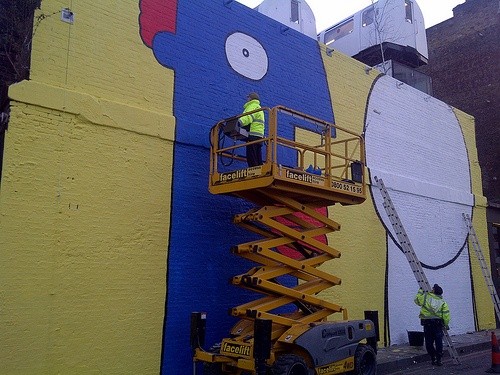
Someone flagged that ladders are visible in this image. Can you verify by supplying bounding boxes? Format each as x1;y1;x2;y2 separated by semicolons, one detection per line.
374;176;462;365
462;213;500;322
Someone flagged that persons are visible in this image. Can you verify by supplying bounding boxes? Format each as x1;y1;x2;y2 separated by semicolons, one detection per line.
237;93;265;167
413;284;451;366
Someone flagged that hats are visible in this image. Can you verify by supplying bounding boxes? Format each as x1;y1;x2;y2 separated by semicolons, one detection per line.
434;284;443;295
247;91;258;99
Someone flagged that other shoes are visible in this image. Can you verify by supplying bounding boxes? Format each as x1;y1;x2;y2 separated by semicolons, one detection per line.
432;357;444;365
